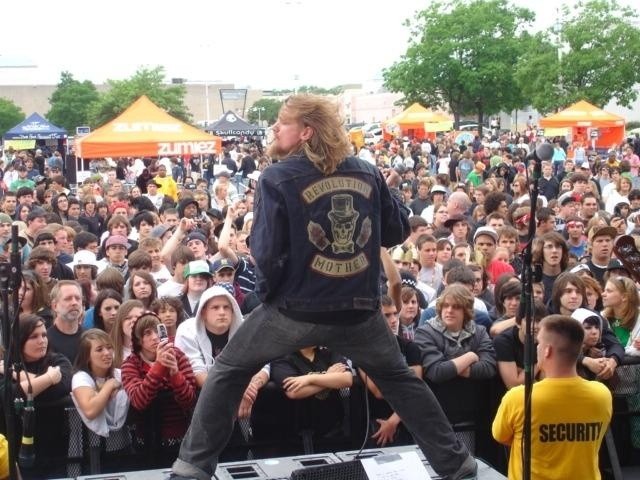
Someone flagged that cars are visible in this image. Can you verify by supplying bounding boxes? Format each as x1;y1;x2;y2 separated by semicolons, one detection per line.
359;118;390;149
454;120;495;135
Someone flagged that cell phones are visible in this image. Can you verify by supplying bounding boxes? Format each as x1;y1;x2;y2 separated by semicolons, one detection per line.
158;323;169;343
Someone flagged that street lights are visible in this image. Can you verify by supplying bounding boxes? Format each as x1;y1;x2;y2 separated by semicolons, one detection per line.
251;104;266;127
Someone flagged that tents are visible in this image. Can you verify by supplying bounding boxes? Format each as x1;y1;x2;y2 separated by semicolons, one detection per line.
530;101;629;154
9;107;69;143
74;97;216;162
375;99;458;142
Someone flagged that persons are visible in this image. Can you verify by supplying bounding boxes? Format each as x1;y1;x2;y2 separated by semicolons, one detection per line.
166;95;482;479
1;116;639;440
490;312;611;479
0;313;70;479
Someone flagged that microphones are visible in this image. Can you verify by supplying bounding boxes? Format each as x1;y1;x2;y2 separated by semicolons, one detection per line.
19;386;36;467
526;143;554;160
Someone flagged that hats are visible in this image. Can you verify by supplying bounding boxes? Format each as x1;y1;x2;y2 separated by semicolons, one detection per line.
472;225;499;242
179;197;199;216
588;223;617;243
183;259;214;278
185;231;208;244
104;235;128;251
71;250;99;269
211;258;235;273
